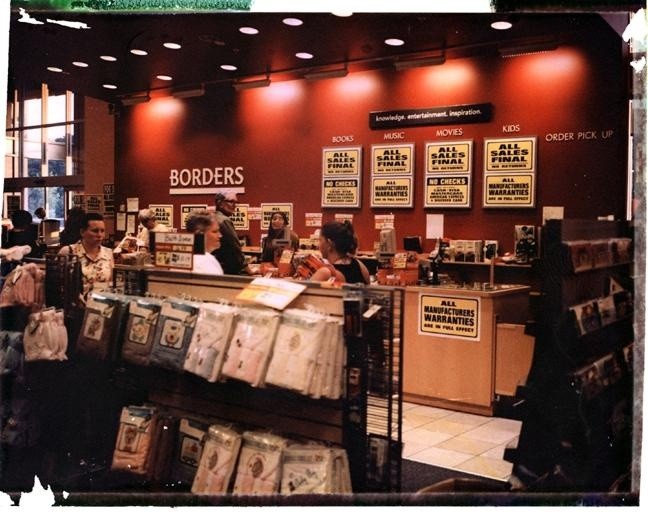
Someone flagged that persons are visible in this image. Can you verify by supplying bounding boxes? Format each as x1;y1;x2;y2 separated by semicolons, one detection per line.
34;209;50;224
181;208;225;274
310;217;371;287
260;209;299;263
137;206;170;244
52;213;114;293
212;188;254;275
9;208;45;260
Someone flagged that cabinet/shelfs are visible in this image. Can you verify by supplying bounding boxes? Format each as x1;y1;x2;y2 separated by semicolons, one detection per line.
502;216;635;493
